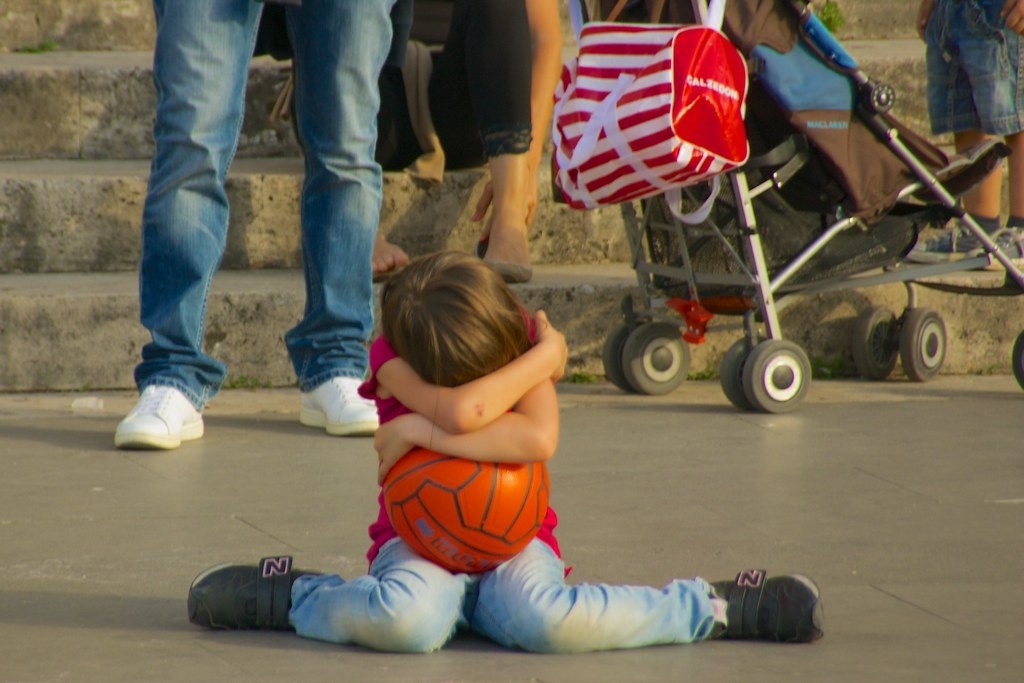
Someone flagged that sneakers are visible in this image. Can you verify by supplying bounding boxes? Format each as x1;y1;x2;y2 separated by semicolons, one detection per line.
297;373;380;438
906;219;989;263
113;384;205;449
982;225;1024;272
710;568;826;644
190;552;321;636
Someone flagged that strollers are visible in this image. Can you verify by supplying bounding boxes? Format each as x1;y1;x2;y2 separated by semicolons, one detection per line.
570;0;1024;415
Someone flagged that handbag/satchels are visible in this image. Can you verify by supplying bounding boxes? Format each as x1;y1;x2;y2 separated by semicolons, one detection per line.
549;23;749;225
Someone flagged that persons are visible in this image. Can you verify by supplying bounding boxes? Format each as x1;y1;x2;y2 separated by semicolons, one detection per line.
270;0;561;281
906;0;1024;271
187;250;824;652
113;1;394;450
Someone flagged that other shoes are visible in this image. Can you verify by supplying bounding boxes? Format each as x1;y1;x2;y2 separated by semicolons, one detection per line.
483;254;532;284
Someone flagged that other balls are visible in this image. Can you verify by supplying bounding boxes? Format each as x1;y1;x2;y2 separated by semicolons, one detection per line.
382;445;550;574
